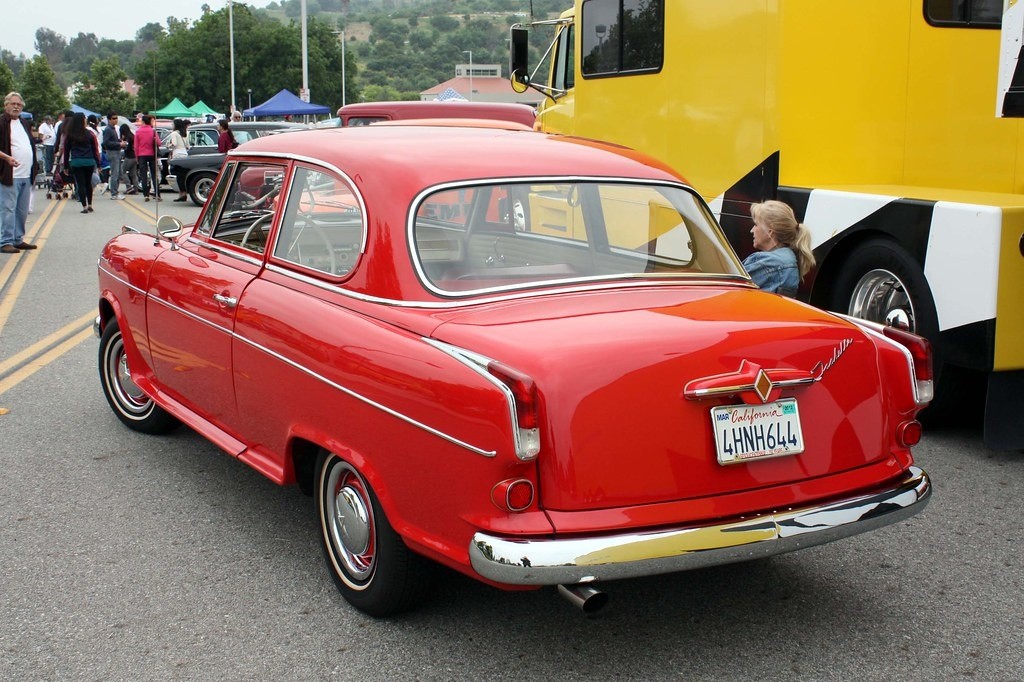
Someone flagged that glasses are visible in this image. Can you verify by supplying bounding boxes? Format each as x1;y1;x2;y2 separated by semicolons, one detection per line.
111;117;118;120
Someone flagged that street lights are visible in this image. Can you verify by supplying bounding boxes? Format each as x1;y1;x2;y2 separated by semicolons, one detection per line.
462;50;472;99
331;31;346;107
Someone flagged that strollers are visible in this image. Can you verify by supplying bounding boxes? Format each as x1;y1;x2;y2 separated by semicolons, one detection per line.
47;159;76;201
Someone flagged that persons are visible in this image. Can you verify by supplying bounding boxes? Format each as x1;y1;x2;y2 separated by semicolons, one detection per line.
21;91;293;213
688;199;815;301
0;92;37;254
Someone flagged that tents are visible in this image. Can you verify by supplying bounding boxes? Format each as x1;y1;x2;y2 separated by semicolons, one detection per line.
20;88;333;125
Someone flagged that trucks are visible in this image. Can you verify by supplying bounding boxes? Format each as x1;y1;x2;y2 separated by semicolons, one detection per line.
509;0;1023;439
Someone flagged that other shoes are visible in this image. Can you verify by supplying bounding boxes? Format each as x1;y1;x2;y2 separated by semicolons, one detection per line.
101;183;108;195
145;196;150;202
80;209;88;214
156;197;162;201
124;188;134;194
14;242;37;250
1;245;20;253
172;194;187;201
118;194;125;200
88;207;94;212
132;190;139;195
110;194;118;201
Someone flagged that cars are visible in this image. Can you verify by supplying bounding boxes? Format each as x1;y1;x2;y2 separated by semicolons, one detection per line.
94;120;933;620
127;99;535;231
